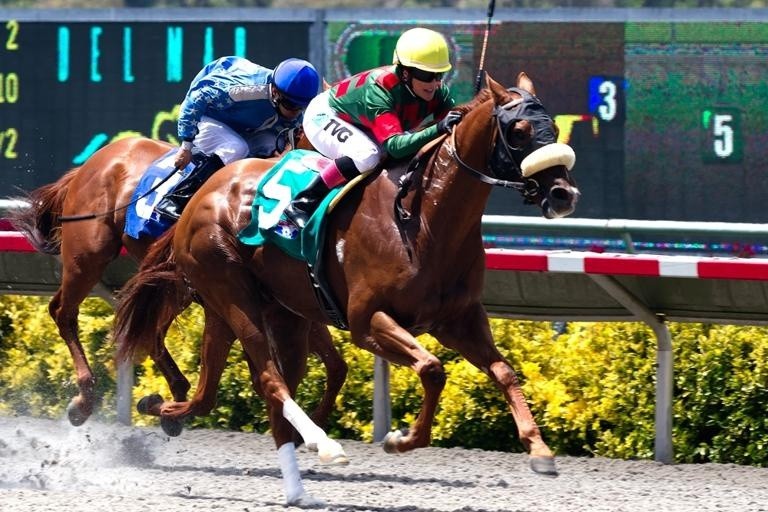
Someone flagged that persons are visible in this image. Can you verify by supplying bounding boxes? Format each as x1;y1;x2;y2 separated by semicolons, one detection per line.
283;28;462;231
154;56;319;220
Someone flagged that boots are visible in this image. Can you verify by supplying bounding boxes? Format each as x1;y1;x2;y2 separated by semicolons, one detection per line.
153;152;225;220
283;158;348;231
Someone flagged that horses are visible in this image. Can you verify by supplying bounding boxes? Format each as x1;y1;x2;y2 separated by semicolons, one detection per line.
106;70;582;508
0;76;348;450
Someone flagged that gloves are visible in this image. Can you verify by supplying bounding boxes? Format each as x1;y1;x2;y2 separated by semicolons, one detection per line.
437;110;462;134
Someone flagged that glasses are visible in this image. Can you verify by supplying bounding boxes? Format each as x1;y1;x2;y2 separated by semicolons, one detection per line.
279;93;301;111
407;68;443;82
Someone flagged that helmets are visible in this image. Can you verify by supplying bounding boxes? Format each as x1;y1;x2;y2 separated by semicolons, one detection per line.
392;28;452;73
272;58;323;105
